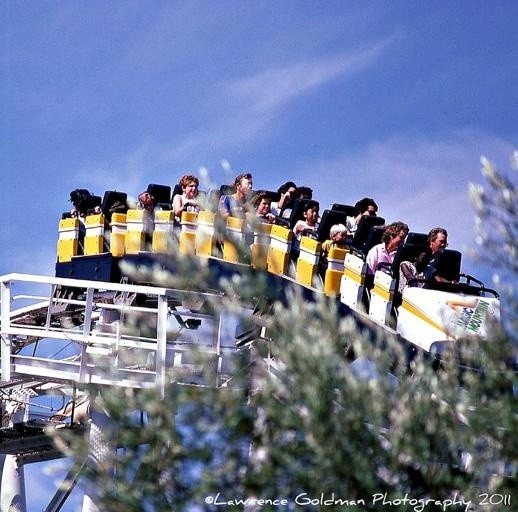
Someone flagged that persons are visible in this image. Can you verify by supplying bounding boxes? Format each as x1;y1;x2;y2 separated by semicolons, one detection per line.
70;173;459;295
